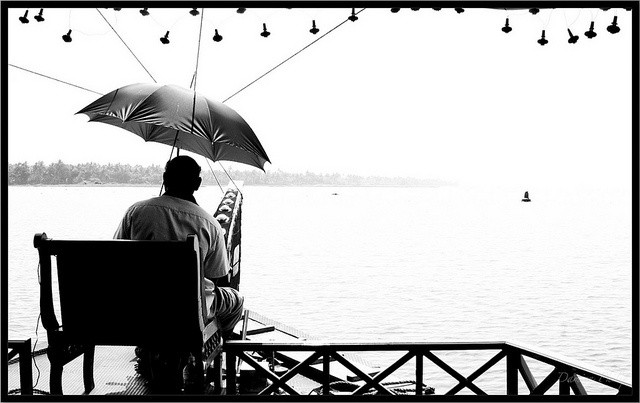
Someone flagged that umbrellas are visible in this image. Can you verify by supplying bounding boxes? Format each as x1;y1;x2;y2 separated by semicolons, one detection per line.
74;70;272;197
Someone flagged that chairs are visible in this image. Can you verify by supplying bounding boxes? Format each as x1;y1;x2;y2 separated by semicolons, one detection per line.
33;229;226;397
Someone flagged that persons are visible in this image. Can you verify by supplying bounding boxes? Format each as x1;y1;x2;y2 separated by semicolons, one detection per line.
112;155;244;330
